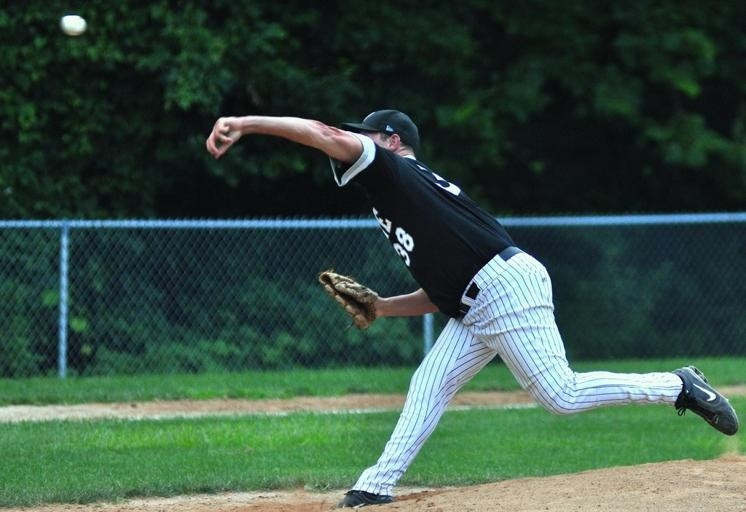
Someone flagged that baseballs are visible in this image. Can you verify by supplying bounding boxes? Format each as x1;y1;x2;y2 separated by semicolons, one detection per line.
58;14;87;35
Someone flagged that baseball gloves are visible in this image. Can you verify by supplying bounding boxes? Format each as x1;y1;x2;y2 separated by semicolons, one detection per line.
317;271;378;330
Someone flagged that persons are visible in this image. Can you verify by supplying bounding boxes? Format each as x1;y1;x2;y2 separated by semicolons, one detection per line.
203;107;742;510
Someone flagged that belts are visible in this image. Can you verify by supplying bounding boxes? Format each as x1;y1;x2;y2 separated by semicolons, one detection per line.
458;246;523;312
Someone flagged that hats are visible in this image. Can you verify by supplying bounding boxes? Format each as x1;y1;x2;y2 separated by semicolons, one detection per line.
341;110;419;145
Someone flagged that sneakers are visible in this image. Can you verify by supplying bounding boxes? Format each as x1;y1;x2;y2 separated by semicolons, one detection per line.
672;365;738;435
338;490;394;508
